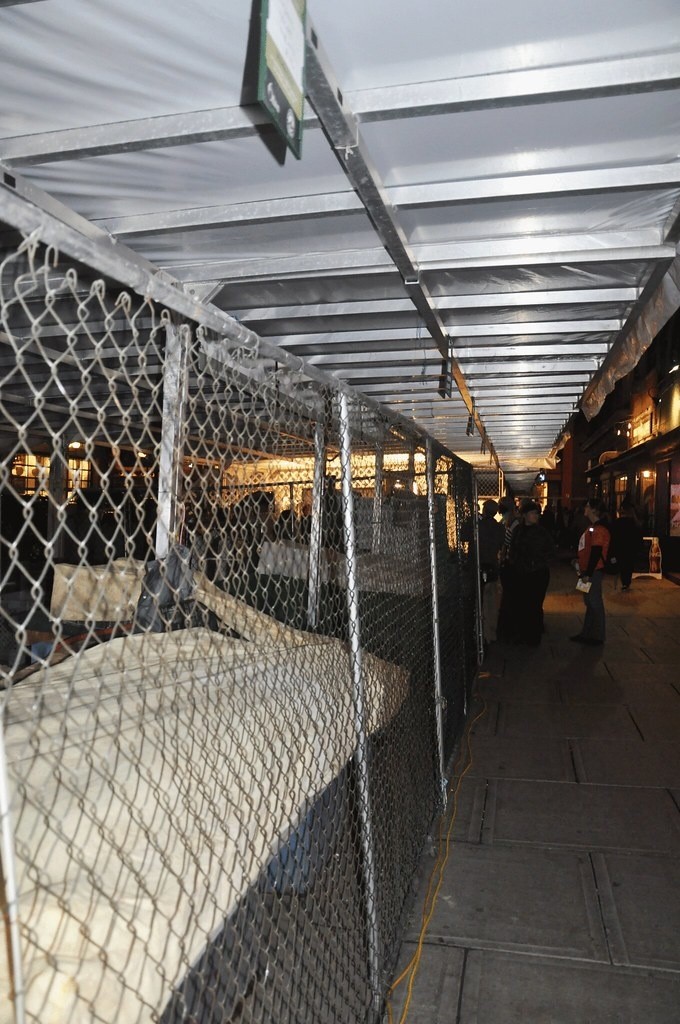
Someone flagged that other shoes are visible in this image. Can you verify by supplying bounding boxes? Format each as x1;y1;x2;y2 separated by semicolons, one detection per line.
621;584;629;593
568;632;605;651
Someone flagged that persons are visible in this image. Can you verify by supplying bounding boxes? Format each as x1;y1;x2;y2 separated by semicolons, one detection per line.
494;495;520;589
614;502;643;592
566;496;612;647
472;499;504;644
536;491;590;555
509;500;554;652
108;426;470;721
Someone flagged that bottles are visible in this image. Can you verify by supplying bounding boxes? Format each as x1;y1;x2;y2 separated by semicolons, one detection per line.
648;536;663;573
